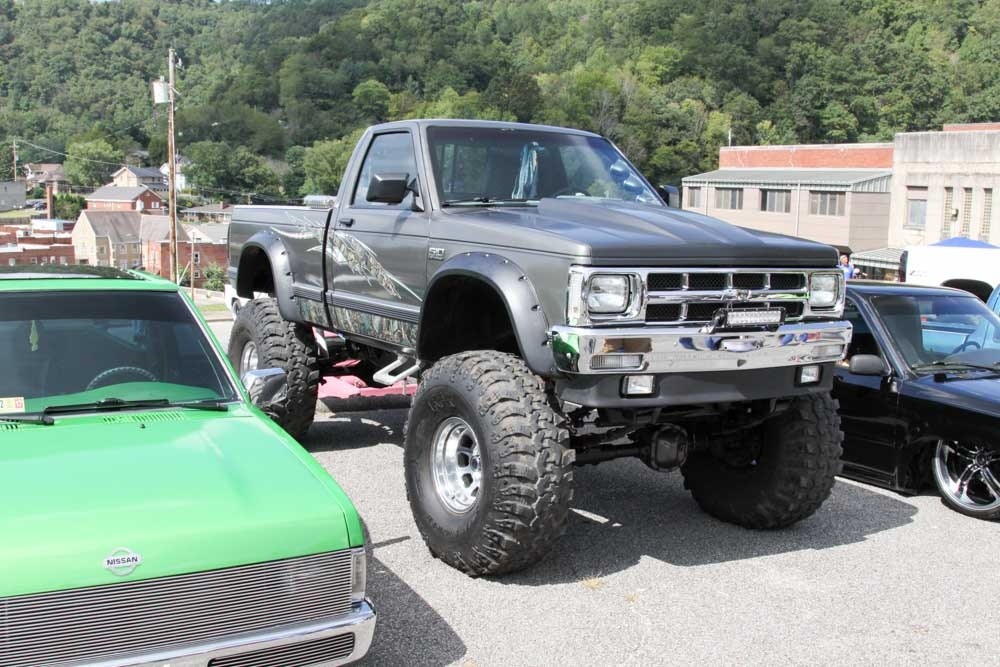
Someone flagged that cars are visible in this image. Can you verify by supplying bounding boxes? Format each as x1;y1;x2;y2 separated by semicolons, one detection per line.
825;281;1000;519
1;262;379;665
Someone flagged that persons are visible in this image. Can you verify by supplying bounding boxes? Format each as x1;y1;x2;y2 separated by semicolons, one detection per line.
840;255;853;280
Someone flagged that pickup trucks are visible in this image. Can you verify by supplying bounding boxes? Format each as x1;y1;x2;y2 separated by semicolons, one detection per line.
920;284;1000;357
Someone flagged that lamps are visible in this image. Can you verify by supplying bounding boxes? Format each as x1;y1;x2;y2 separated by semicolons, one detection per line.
951;208;958;215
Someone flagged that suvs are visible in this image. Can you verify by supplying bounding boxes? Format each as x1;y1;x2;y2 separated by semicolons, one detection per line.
217;116;859;581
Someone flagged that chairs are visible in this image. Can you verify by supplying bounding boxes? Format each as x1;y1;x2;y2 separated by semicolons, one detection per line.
843;317;882;362
38;334;124;396
